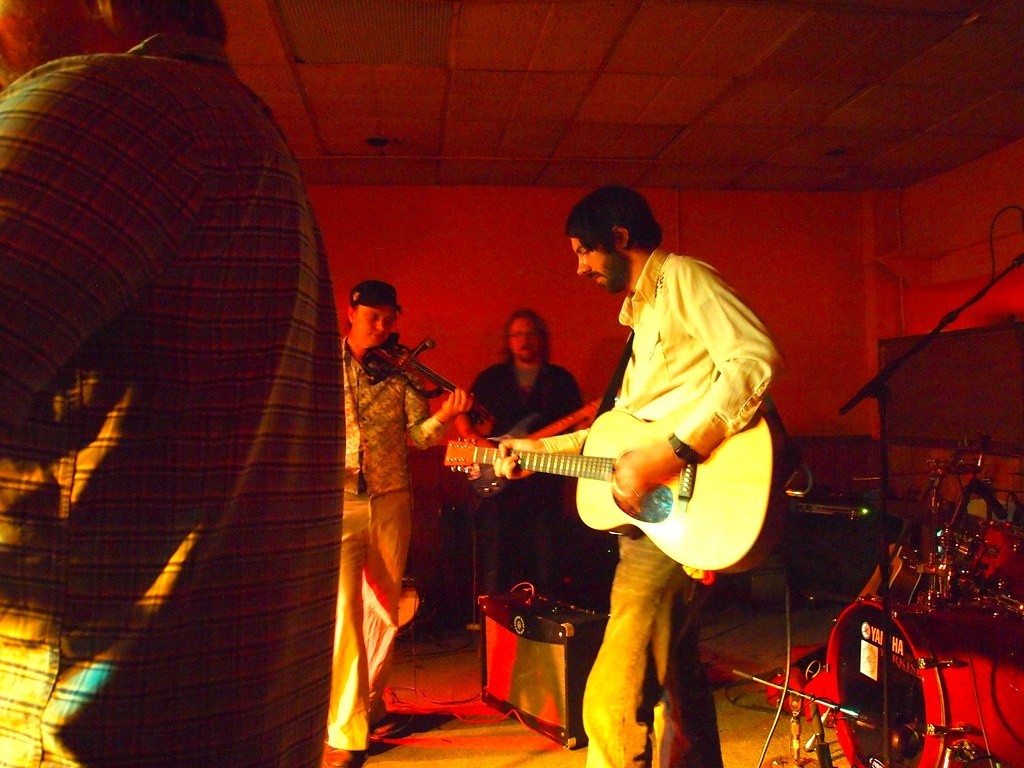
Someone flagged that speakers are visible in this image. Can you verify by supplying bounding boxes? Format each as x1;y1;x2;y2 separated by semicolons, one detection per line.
475;593;608;751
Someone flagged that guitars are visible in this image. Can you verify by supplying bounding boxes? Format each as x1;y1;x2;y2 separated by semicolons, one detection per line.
854;448;955;606
442;397;788;575
464;396;604;499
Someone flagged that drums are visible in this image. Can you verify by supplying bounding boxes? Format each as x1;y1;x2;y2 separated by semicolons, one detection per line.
822;511;1023;768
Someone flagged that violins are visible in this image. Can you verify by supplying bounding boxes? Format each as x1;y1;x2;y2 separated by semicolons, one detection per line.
370;341;496;437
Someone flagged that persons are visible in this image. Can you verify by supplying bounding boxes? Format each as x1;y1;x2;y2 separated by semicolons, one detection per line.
456;309;584;592
318;279;475;768
492;186;784;768
1;0;349;768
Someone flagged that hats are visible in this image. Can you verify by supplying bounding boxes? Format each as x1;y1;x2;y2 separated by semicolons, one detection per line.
350;281;402;312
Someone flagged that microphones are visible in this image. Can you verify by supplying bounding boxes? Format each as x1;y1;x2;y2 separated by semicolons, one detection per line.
887;723;920;760
977;482;1009;520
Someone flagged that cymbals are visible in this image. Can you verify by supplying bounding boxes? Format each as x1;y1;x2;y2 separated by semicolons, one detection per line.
951;445;1023;460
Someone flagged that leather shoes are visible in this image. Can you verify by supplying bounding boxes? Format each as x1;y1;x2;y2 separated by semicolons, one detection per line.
324;743;357;768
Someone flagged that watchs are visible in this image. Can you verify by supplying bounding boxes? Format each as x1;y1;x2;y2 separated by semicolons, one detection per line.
667;433;699;464
